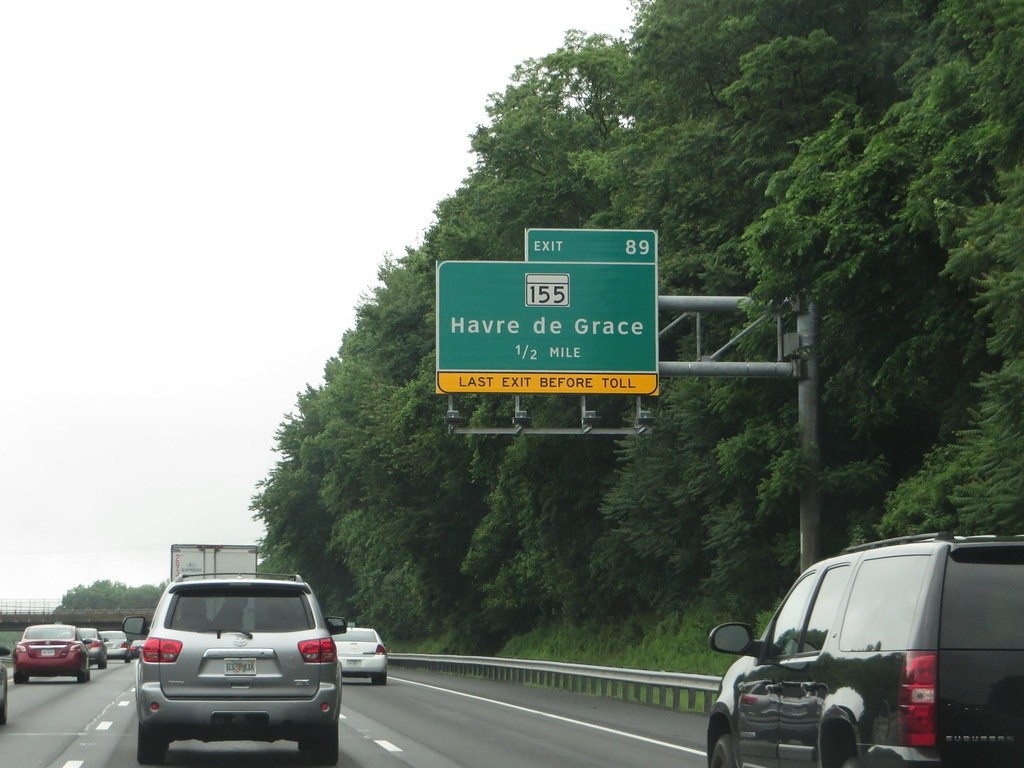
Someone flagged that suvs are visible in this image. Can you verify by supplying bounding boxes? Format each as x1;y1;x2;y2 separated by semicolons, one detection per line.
701;527;1024;768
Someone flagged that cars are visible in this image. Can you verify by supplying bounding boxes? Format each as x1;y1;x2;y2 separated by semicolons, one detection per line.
97;630;129;661
122;572;345;767
125;640;148;664
330;626;387;686
11;624;89;684
0;645;11;726
74;627;110;669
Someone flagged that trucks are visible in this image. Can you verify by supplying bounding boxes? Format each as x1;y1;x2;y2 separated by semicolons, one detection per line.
170;543;258;587
